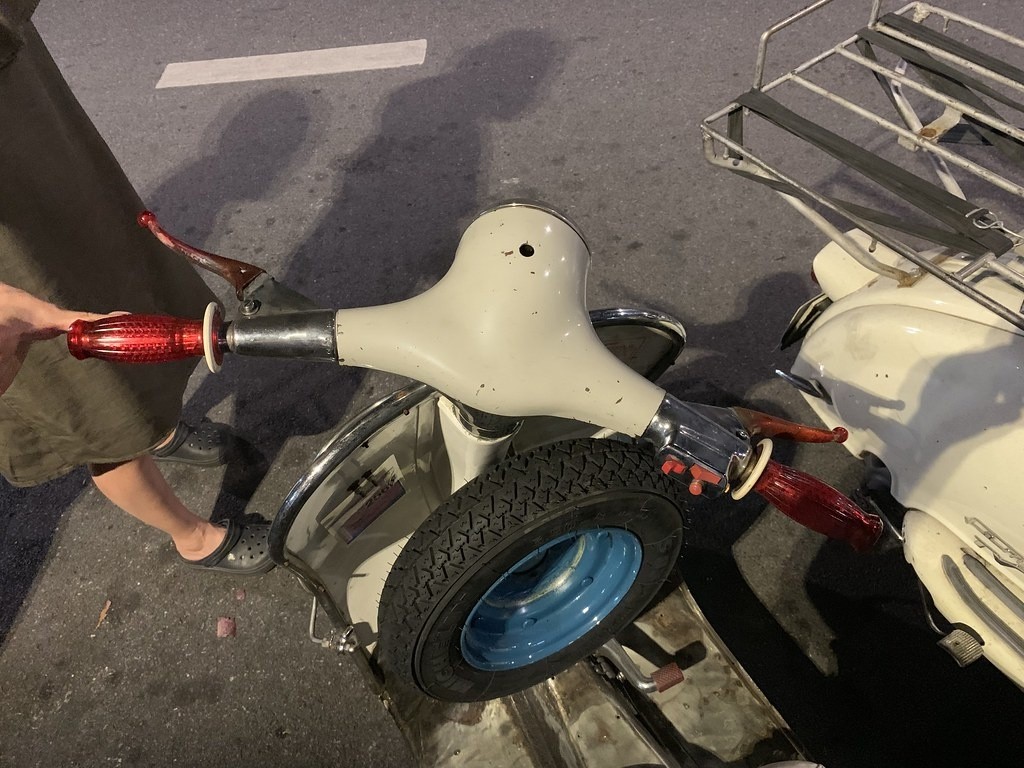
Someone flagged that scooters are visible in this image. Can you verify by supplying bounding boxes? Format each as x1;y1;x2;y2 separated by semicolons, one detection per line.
65;204;888;768
698;0;1024;692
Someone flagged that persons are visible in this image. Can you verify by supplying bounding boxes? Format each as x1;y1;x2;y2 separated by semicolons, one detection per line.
0;0;277;576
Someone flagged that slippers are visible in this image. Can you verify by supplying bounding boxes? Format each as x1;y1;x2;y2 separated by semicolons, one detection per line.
147;419;233;468
177;518;278;574
